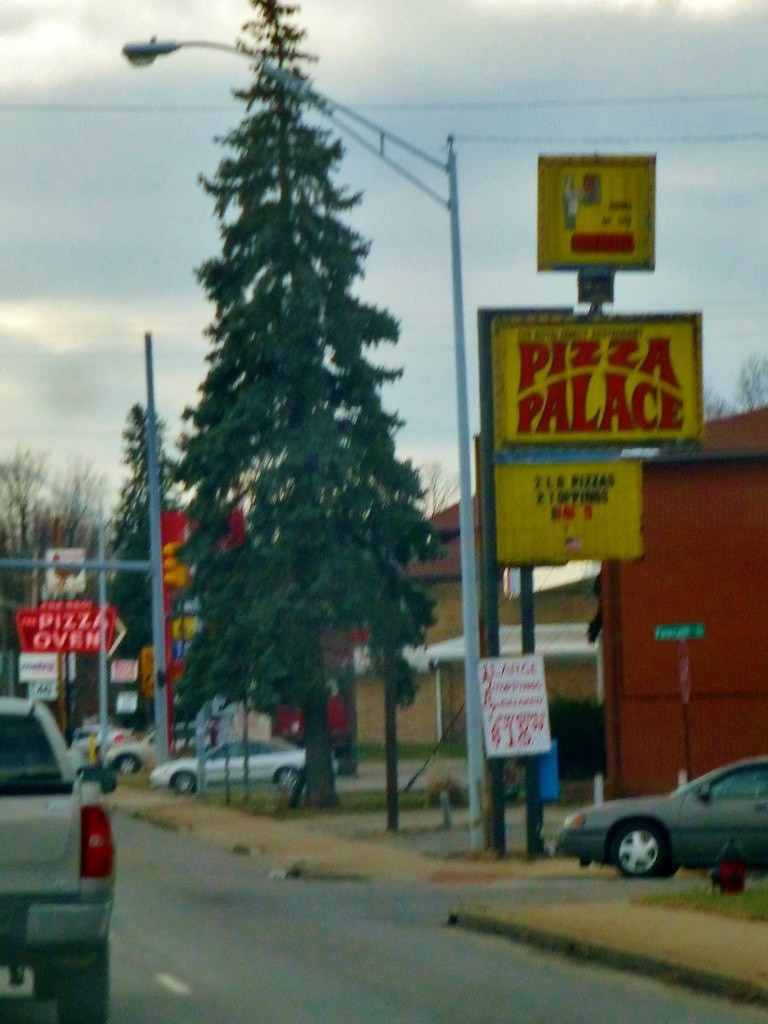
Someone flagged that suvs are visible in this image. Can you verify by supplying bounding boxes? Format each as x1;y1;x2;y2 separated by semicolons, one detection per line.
0;696;118;1024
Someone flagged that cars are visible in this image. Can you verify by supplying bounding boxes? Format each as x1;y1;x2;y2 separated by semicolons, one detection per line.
103;728;215;778
560;754;768;883
148;735;339;799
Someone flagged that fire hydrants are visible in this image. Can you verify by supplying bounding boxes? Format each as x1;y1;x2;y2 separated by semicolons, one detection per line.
709;836;751;897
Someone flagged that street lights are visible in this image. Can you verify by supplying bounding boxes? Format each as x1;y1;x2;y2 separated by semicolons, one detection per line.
122;37;487;854
